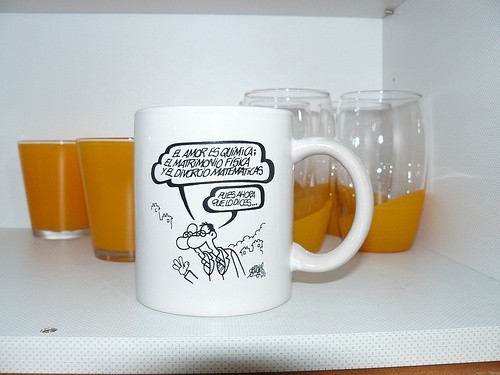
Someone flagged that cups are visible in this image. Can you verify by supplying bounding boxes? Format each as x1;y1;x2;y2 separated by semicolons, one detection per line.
326;98;397;239
132;106;374;317
16;140;90;240
340;86;428;254
241;89;336;272
74;138;136;263
